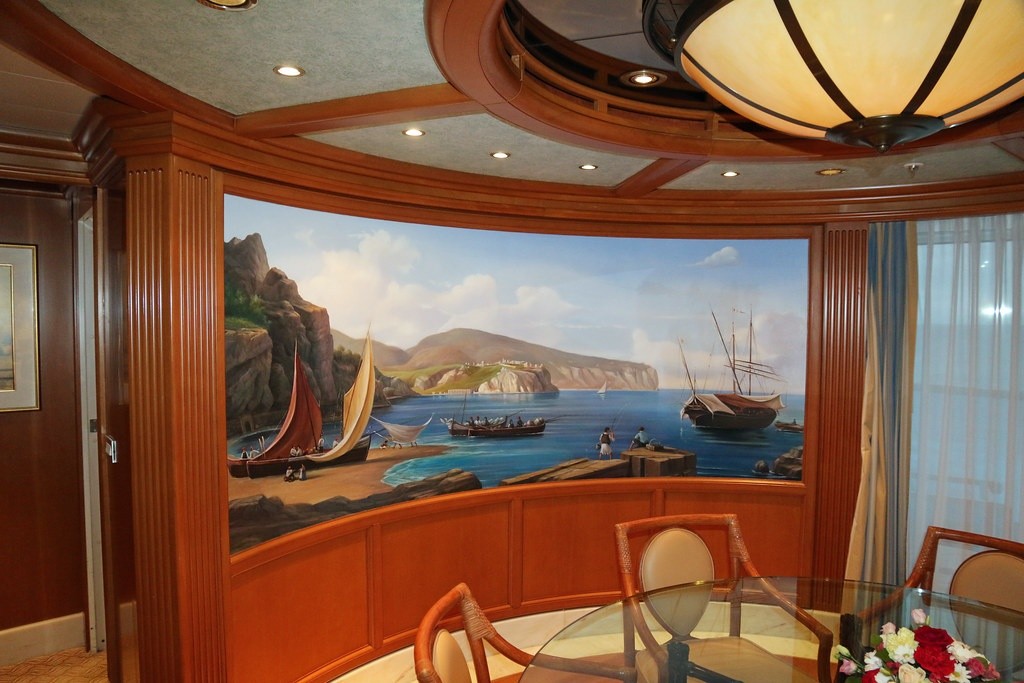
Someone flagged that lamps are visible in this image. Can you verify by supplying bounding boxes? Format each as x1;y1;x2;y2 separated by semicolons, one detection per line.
680;0;1024;148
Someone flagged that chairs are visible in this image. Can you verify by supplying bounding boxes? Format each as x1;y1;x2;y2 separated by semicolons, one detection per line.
840;526;1022;678
414;581;636;682
613;514;833;682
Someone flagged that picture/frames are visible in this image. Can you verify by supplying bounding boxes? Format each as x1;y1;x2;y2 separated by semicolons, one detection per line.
0;243;41;414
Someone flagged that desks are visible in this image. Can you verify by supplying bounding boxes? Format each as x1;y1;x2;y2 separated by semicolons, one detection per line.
519;577;1023;682
